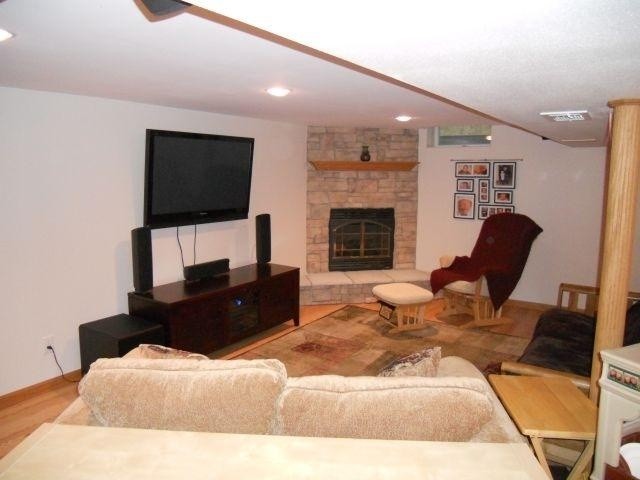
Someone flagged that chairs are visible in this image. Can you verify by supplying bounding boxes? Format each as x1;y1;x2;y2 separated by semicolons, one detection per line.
493;270;639;392
431;213;543;325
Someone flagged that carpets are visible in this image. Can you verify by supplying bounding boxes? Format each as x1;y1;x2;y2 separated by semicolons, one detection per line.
217;301;531;380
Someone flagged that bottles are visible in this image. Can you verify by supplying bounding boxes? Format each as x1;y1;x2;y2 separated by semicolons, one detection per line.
361;145;370;161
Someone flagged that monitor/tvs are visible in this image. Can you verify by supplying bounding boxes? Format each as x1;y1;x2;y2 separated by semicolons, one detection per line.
144;128;254;229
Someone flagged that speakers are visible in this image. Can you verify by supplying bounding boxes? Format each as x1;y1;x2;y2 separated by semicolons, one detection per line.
132;227;152;296
184;259;229;281
256;214;271;265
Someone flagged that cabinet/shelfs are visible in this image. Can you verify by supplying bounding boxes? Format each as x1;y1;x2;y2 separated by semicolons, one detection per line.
127;269;301;356
79;313;165;380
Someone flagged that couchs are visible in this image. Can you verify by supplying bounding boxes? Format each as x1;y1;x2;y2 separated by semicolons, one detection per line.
0;344;551;480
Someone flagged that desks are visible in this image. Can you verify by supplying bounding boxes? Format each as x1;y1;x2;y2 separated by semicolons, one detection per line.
488;373;598;480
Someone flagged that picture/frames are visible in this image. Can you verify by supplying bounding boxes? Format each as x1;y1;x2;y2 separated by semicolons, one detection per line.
452;159;518;222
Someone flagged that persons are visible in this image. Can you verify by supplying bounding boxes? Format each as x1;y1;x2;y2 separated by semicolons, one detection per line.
458;164;511;217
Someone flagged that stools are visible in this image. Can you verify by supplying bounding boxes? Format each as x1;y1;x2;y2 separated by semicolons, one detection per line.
372;283;434;334
